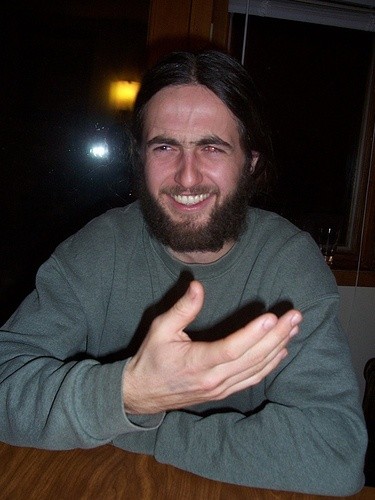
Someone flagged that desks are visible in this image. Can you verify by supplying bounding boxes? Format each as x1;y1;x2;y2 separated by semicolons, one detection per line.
0;440;375;500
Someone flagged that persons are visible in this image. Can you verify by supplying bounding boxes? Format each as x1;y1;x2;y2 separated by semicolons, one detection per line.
0;49;369;496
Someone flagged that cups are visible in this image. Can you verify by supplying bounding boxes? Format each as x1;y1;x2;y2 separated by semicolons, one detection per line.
317;227;340;264
